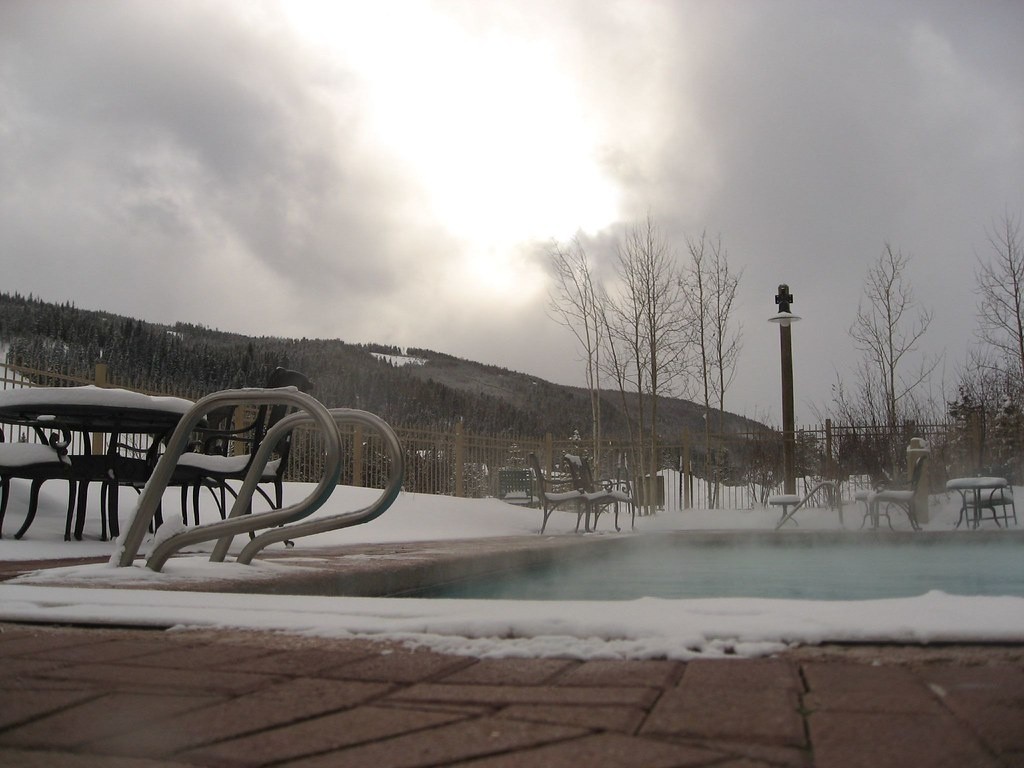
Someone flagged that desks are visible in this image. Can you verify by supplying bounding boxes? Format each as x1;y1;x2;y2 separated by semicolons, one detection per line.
0;402;208;541
854;496;897;531
947;485;1006;529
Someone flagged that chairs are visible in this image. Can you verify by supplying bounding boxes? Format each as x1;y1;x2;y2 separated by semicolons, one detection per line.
563;453;636;533
0;366;312;548
528;454;592;535
974;481;1017;527
870;455;925;532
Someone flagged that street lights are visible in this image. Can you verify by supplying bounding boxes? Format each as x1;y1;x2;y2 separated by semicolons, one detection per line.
768;311;803;494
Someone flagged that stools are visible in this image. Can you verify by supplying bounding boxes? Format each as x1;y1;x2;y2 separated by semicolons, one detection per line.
769;500;801;527
